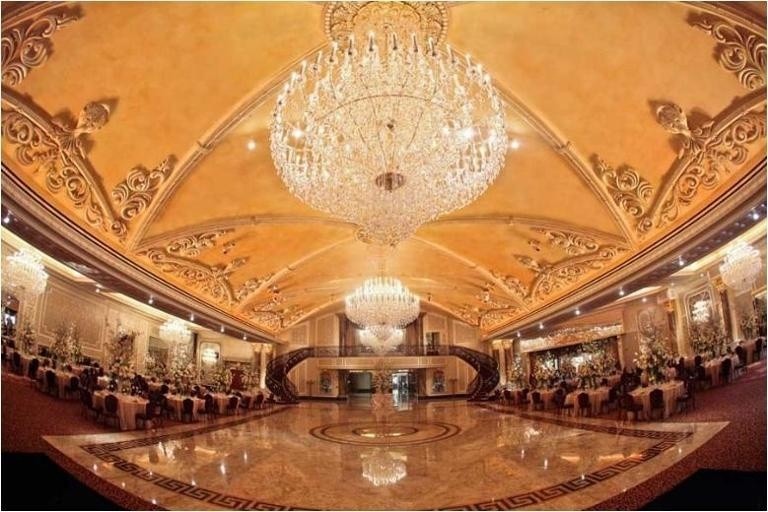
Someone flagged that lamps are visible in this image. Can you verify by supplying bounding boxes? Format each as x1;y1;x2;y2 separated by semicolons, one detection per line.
159;316;191;346
691;299;711;323
718;241;763;295
1;249;47;301
355;326;407;355
270;2;509;250
361;446;409;487
343;249;422;326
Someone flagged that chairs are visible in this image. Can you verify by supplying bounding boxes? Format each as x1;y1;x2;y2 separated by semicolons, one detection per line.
493;338;762;416
0;339;274;431
